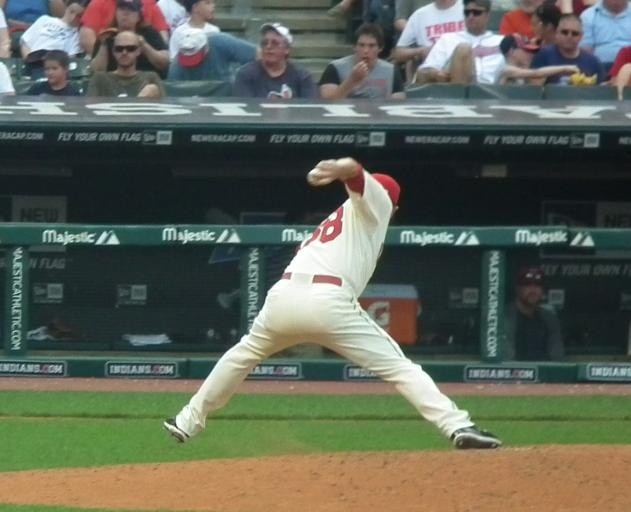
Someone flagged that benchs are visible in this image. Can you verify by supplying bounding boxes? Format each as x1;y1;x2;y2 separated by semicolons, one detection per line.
0;0;629;98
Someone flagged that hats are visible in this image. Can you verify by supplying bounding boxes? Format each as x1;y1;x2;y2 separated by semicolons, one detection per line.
174;26;210;66
262;21;294;49
517;267;547;283
371;173;401;205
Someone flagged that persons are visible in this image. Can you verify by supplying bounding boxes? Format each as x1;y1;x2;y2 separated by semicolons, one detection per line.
327;1;631;99
232;23;314;100
316;22;408;100
502;263;565;362
164;157;502;449
1;0;262;100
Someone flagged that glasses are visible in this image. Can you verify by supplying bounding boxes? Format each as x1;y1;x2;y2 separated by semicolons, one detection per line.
560;27;582;35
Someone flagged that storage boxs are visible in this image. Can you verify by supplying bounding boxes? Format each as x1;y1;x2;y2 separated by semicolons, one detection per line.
357;283;422;345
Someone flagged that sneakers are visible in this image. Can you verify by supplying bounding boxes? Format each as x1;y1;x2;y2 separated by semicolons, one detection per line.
165;417;189;443
456;426;500;447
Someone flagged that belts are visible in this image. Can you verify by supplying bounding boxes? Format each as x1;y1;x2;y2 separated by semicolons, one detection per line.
281;272;344;286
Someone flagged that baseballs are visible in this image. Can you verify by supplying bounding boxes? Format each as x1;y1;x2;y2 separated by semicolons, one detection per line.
308;169;322;186
